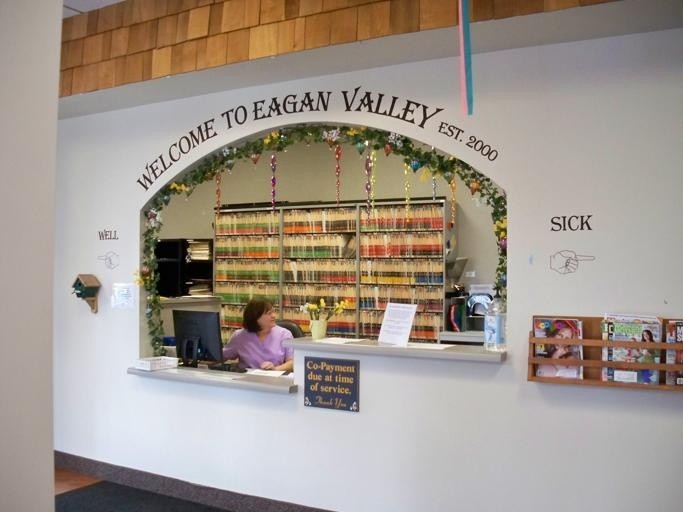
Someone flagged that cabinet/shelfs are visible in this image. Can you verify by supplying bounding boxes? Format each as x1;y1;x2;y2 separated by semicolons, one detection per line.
527;314;683;392
212;197;457;347
153;238;214;297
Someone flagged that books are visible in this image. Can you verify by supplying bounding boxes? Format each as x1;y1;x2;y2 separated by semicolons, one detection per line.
187;240;212;260
187;274;213;296
601;313;683;386
533;317;583;380
215;204;444;343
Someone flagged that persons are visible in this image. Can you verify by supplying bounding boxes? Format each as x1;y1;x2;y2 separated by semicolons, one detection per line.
536;321;581;379
636;330;656;383
220;299;293;370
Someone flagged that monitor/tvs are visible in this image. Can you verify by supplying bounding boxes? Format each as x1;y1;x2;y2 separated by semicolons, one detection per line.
173;310;223;368
